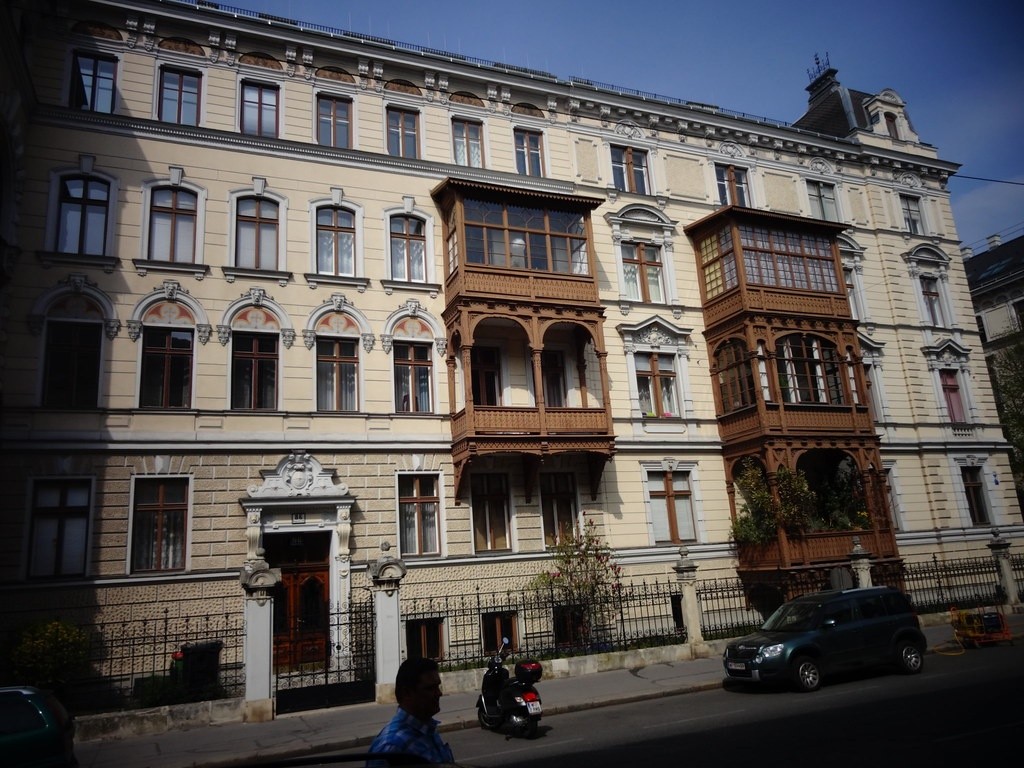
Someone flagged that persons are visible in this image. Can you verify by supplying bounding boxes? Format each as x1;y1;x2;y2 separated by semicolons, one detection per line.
361;657;455;768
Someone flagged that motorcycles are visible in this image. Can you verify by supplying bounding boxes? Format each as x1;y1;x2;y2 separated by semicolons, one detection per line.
474;635;542;742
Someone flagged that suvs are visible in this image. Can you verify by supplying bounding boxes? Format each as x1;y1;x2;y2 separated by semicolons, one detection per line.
722;583;927;693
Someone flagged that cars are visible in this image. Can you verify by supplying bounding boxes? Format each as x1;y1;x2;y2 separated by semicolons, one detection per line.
1;686;77;767
